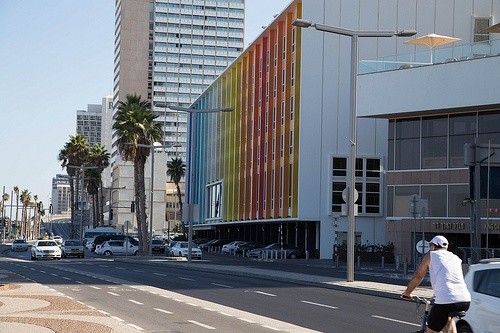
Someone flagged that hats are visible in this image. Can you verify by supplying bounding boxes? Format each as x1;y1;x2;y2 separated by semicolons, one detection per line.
427;235;448;249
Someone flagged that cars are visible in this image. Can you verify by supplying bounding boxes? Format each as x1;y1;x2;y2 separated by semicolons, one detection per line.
94;240;142;256
247;243;302;260
31;240;62;259
166;241;179;255
192;236;259;254
171;240;203;259
151;238;165;254
54;235;65;244
11;239;28;251
63;237;85;260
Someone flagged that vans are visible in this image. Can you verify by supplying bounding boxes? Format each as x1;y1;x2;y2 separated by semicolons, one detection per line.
83;227;124;247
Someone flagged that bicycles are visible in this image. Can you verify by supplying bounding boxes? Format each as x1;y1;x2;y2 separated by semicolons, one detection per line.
400;292;467;333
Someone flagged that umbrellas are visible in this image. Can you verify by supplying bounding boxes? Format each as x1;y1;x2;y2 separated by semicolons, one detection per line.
479;22;500;33
403;32;462;63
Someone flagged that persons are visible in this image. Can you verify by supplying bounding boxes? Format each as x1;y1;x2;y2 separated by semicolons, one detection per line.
305;249;309;263
401;235;471;333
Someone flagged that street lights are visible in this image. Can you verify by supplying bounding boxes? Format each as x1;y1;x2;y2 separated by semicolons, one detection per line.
291;18;417;283
0;185;9;229
60;164;100;252
125;140;183;258
153;100;233;263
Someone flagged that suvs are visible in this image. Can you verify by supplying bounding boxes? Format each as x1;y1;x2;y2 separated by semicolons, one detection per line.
452;259;500;333
90;231;139;250
